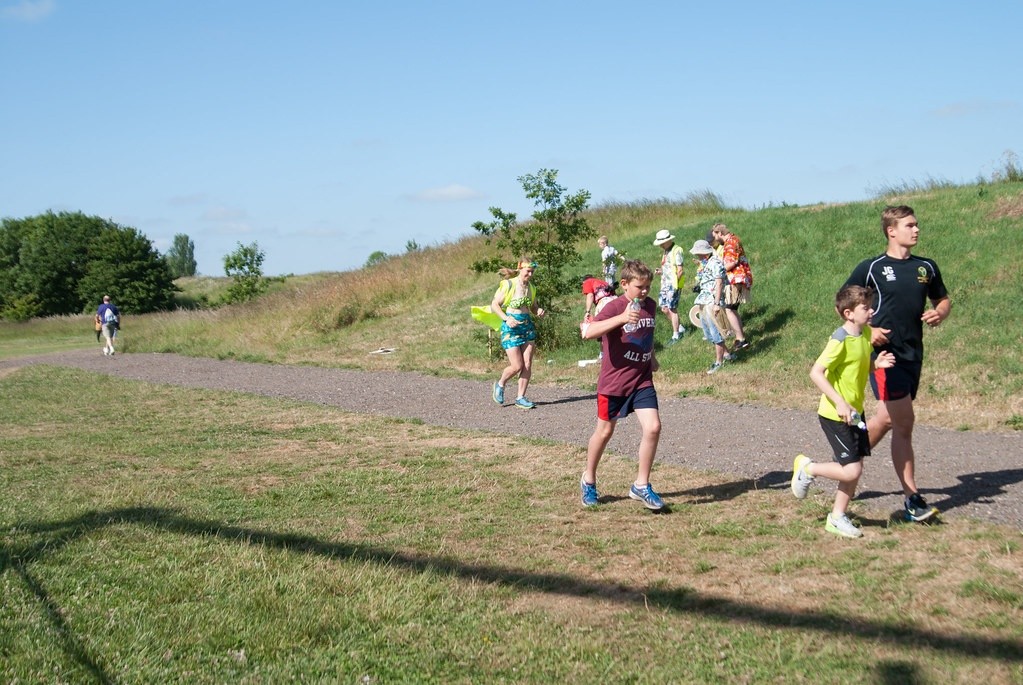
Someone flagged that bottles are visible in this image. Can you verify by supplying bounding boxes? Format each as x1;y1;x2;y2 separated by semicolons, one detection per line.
624;297;641;332
113;328;117;336
850;411;867;432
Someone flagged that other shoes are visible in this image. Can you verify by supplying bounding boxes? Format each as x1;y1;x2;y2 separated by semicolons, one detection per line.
729;340;750;352
677;324;686;334
706;363;726;375
667;333;683;347
724;354;738;362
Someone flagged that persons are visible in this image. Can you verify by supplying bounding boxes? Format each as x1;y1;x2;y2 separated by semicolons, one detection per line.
790;285;895;539
580;258;664;512
95;295;120;356
597;236;629;288
689;223;753;374
834;205;950;522
653;230;687;342
470;258;545;409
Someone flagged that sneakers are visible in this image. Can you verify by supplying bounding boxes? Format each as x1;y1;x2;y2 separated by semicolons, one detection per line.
791;454;816;499
493;380;506;405
825;513;863;540
580;472;600;506
904;493;940;521
515;397;536;410
629;483;665;510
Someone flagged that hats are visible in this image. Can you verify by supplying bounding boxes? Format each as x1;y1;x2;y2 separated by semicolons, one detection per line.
654;230;676;246
689;239;714;255
